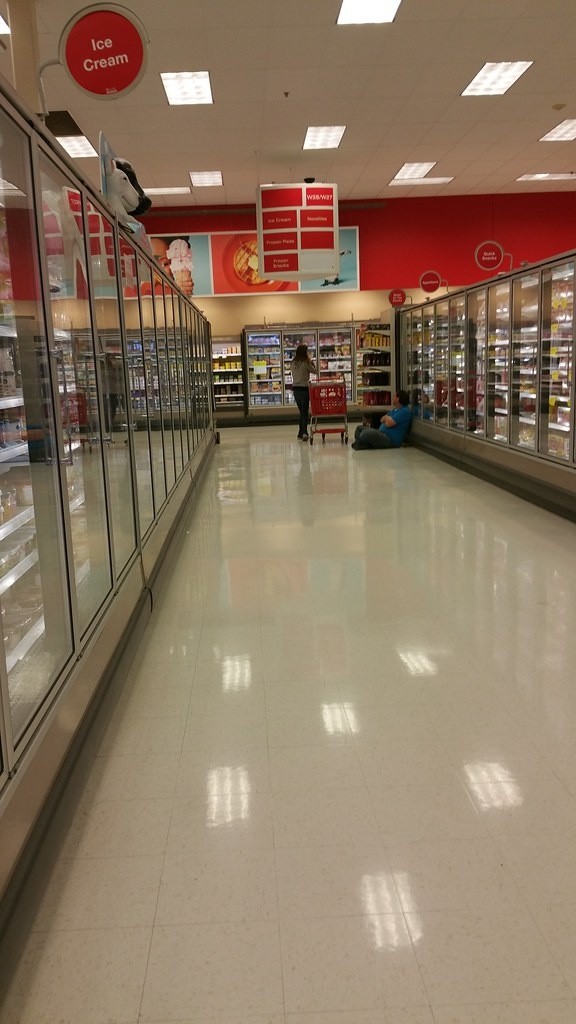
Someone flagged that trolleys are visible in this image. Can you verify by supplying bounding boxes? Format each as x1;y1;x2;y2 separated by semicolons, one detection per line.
305;372;349;445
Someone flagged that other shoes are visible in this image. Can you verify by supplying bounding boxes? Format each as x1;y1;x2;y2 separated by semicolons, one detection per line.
297;436;302;439
352;443;356;449
302;433;308;441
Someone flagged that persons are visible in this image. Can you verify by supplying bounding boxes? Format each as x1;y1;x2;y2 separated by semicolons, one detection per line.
351;390;412;451
0;406;53;462
290;344;319;441
99;357;119;433
411;388;431;420
150;236;194;295
